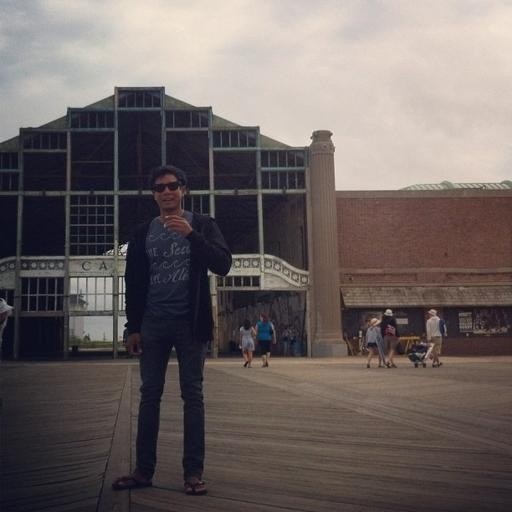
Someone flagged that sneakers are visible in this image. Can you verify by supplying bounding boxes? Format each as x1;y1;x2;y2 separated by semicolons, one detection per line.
432;362;444;368
367;359;398;369
244;359;269;368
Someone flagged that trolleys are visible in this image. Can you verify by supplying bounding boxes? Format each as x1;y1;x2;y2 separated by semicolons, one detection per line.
409;341;435;369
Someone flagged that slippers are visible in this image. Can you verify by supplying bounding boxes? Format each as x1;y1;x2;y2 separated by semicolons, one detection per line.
183;477;207;495
111;476;153;490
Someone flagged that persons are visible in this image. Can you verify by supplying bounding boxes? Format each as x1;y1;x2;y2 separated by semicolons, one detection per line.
426;309;447;368
110;163;234;496
122;322;133;359
281;324;301;358
254;312;277;368
380;309;401;369
239;319;257;368
364;318;383;369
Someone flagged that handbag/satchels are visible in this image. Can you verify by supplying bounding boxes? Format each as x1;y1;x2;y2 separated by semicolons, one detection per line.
270;326;273;335
384;324;396;336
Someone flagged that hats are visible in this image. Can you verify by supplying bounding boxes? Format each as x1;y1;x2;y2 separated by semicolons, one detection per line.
371;318;381;326
428;309;437;316
384;309;393;316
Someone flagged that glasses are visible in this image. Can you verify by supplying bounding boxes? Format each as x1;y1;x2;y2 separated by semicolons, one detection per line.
153;182;182;193
